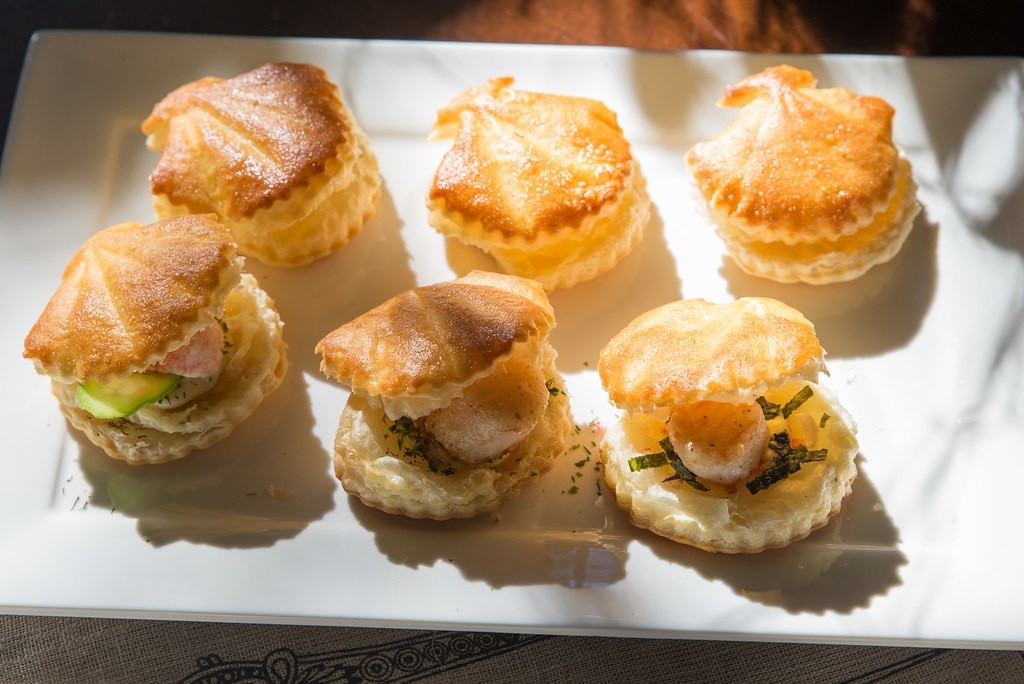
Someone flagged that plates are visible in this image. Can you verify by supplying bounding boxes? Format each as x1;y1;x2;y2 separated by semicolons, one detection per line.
0;27;1024;651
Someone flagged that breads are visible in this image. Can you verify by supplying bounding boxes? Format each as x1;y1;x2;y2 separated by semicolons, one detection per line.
22;213;286;464
315;270;566;518
424;76;648;287
139;62;381;268
688;64;917;287
599;295;859;556
